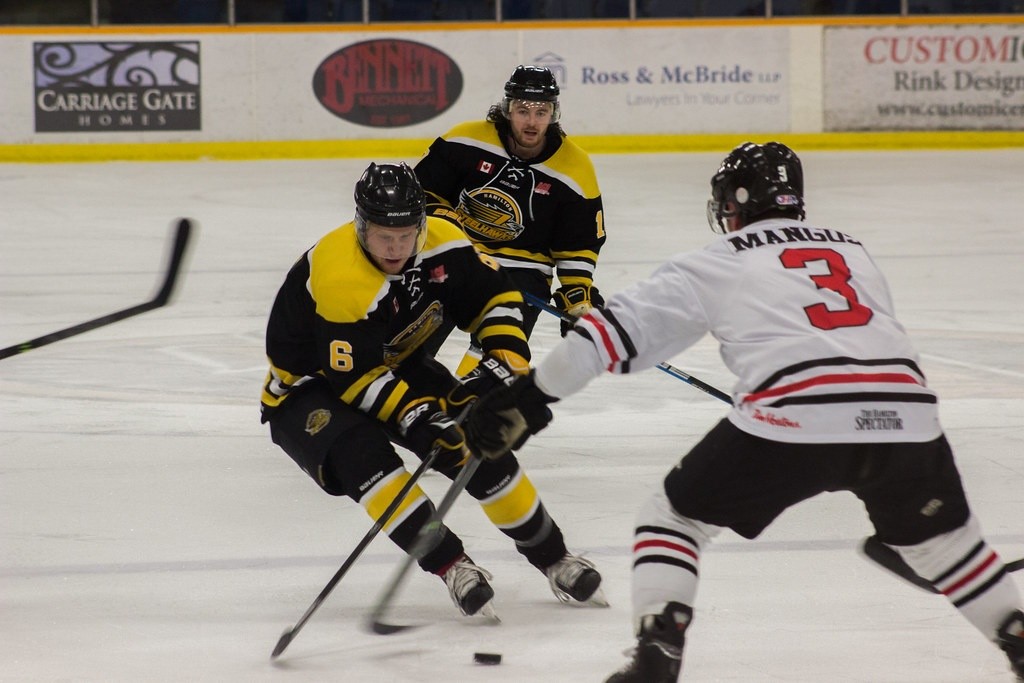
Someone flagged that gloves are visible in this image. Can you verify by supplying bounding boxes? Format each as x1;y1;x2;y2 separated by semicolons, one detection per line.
396;399;470;467
553;284;606;338
444;354;516;412
465;374;553;458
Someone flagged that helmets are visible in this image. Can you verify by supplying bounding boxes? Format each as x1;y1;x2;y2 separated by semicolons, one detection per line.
504;64;560;118
354;161;425;228
712;140;805;217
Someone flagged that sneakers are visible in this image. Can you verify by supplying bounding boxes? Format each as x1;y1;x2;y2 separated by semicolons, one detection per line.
995;609;1024;683
441;553;503;625
541;550;610;609
603;599;694;683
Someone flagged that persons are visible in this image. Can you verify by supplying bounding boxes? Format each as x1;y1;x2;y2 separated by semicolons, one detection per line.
457;141;1024;683
260;64;607;615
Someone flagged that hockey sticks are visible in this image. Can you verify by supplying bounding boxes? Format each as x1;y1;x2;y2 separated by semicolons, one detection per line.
364;450;485;637
518;290;739;409
860;532;1024;598
267;396;476;663
1;215;195;362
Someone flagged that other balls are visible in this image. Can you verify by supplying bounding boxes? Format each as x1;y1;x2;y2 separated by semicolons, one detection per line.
473;652;503;665
